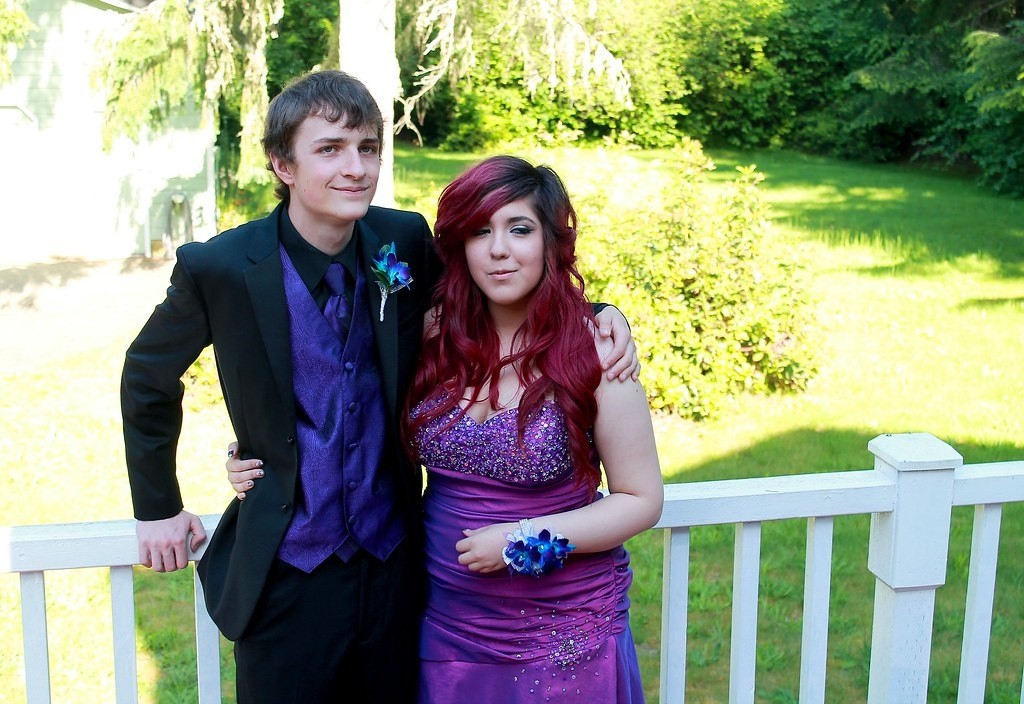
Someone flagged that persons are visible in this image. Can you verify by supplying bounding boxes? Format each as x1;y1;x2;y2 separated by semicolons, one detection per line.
226;155;664;704
120;71;642;704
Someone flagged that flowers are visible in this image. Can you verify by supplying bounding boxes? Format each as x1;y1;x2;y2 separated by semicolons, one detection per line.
370;241;413;322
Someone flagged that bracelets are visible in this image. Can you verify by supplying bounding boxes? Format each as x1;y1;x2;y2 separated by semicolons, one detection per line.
503;519;576;577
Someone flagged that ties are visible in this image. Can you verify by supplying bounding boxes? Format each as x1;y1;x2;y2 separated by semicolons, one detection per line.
323;265;362;564
